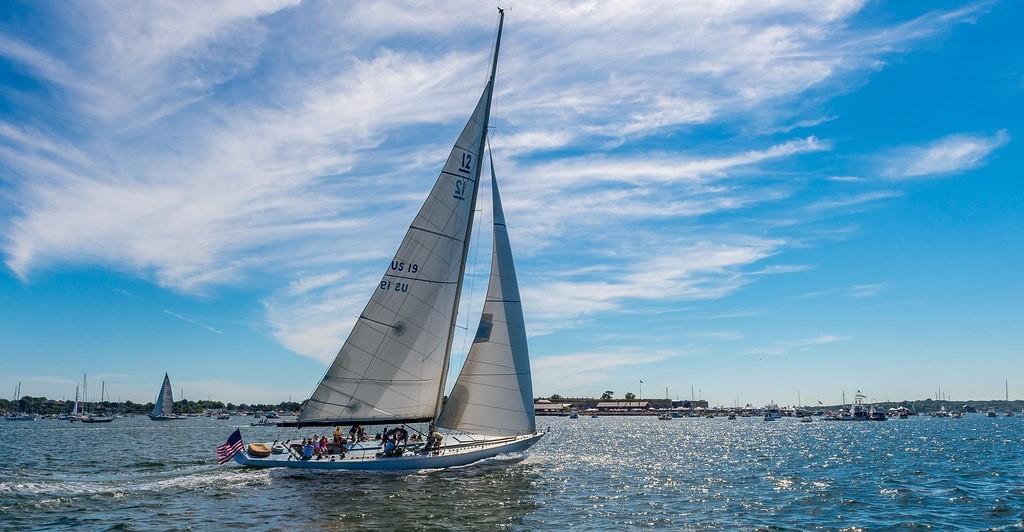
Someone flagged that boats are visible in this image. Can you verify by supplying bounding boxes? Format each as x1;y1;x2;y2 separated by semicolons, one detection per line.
570;383;1018;423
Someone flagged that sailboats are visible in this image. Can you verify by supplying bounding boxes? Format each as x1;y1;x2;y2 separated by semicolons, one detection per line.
216;5;552;470
6;372;115;422
151;371;177;420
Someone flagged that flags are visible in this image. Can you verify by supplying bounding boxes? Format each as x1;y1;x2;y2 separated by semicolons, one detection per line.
216;428;244;466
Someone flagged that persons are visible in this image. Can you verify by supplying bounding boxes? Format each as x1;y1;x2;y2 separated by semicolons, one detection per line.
300;423;443;460
257;417;270;425
